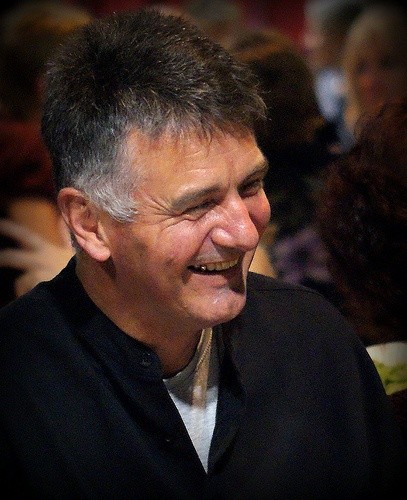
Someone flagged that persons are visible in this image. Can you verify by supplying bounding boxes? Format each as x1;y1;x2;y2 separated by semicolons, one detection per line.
0;9;407;500
0;0;406;412
